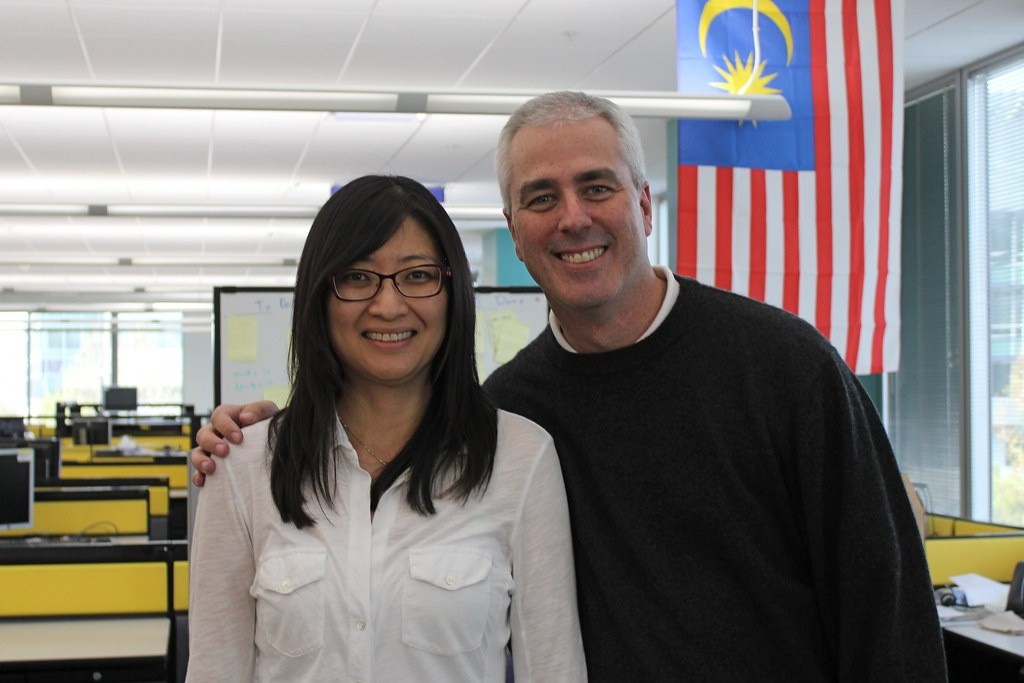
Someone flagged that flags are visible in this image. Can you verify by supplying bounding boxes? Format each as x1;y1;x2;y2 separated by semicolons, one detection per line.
677;0;904;376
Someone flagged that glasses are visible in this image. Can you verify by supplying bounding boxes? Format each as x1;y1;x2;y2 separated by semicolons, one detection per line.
327;262;451;302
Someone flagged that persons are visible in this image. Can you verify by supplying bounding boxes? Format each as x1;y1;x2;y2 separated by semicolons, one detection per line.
185;174;588;683
191;91;948;682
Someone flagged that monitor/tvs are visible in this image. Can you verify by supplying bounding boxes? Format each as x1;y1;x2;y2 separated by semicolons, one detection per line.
105;388;137;410
0;417;111;530
